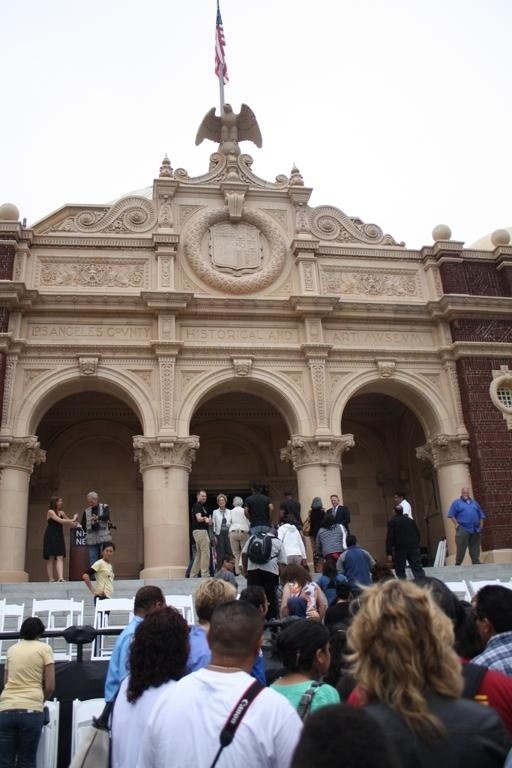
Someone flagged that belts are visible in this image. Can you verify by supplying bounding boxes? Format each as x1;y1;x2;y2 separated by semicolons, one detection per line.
232;530;244;533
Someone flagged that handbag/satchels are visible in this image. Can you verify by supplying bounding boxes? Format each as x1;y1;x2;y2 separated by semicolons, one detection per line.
303;510;311;536
67;687;114;768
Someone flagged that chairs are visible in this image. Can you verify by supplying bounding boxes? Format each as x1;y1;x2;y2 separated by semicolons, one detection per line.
468;581;505;597
0;598;24;663
93;597;135;664
165;594;196;629
446;581;472;604
32;599;85;663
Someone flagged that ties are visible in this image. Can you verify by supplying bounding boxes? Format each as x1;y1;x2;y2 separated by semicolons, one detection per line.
333;508;336;516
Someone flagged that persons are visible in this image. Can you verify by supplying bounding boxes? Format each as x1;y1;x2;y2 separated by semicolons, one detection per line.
447;488;486;566
383;506;427;580
327;621;349;667
211;494;231;572
313;511;347;577
449;599;485;664
290;579;510;768
238;527;286;603
185;576;237;679
306;497;327;572
457;585;511;674
333;535;376;588
239;586;266;686
348;574;512;736
81;542;114;657
327;494;351;541
226;496;250;575
42;495;78;582
275;512;308;585
268;619;341;720
277;564;327;622
279;490;303;533
213;551;240;587
244;482;275;533
133;598;304;768
99;585;167;722
184;506;215;579
109;606;195;767
323;581;352;623
189;489;212;579
318;559;348;606
392;492;416;519
76;490;110;582
0;616;55;768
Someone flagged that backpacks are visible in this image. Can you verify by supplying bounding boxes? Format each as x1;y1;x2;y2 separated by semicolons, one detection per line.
247;529;280;566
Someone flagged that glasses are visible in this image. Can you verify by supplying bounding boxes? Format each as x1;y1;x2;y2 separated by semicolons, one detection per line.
224;559;237;569
263;602;270;606
